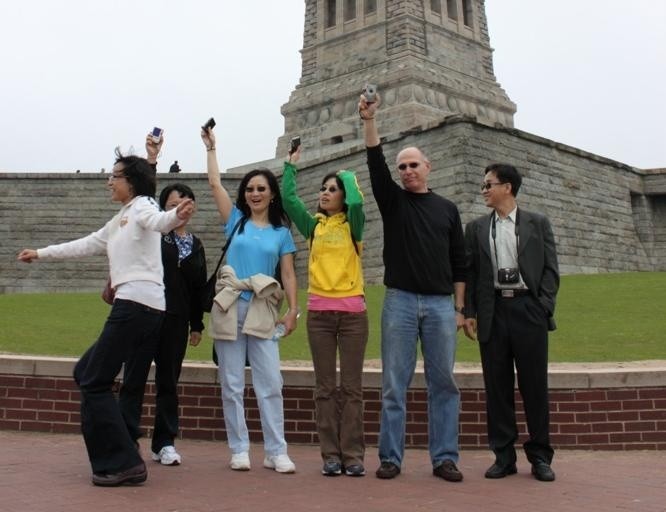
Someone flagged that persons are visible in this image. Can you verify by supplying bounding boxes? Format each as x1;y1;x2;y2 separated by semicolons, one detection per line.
17;146;196;488
117;135;207;467
169;161;181;173
463;164;560;481
357;93;466;482
201;125;299;473
281;146;368;477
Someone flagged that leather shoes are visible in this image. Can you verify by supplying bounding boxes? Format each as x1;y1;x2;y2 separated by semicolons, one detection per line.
485;463;517;478
92;442;147;486
532;460;555;481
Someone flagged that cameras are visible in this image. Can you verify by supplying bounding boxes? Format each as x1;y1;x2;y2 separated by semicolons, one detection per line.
497;267;520;283
365;82;378;102
289;135;301;152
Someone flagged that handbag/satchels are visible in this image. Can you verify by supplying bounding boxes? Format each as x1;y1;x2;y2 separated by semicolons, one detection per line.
201;274;217;312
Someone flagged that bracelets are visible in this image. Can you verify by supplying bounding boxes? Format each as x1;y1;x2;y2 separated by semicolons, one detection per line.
206;147;215;151
361;117;374;120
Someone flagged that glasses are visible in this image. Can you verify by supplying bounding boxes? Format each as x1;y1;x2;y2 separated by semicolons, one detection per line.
111;175;130;181
320;186;335;192
398;162;417;169
245;187;265;192
481;183;504;190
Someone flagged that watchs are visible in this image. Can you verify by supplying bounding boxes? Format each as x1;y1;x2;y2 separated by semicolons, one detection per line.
455;306;468;315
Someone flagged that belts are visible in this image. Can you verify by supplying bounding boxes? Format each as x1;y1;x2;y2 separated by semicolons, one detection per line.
495;289;528;299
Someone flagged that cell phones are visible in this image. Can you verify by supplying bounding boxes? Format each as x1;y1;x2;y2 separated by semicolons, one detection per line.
151;126;163;144
201;117;216;134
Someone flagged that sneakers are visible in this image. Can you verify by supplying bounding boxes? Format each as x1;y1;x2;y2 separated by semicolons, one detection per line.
433;461;462;481
376;462;399;479
231;453;250;470
152;446;181;465
345;464;367;476
264;455;296;473
321;462;342;475
